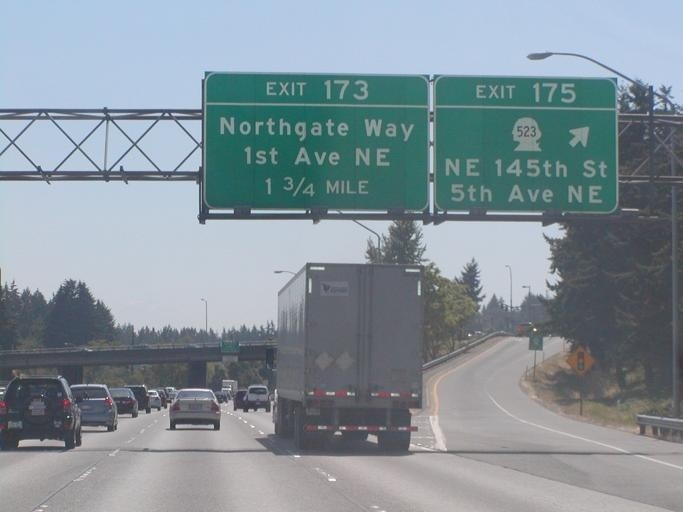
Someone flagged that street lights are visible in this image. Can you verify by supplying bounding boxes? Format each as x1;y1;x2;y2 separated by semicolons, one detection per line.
199;297;207;334
504;264;513;332
529;49;683;418
522;284;530;292
274;269;296;276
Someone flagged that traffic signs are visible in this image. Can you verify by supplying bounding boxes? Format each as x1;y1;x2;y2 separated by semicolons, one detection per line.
221;341;238;352
436;75;618;212
201;70;428;210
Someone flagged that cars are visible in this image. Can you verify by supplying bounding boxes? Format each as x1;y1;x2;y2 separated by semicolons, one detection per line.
127;344;149;349
0;375;270;450
64;347;92;352
517;322;535;337
184;344;200;348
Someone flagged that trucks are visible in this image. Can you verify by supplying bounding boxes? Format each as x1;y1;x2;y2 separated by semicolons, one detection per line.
269;264;426;453
